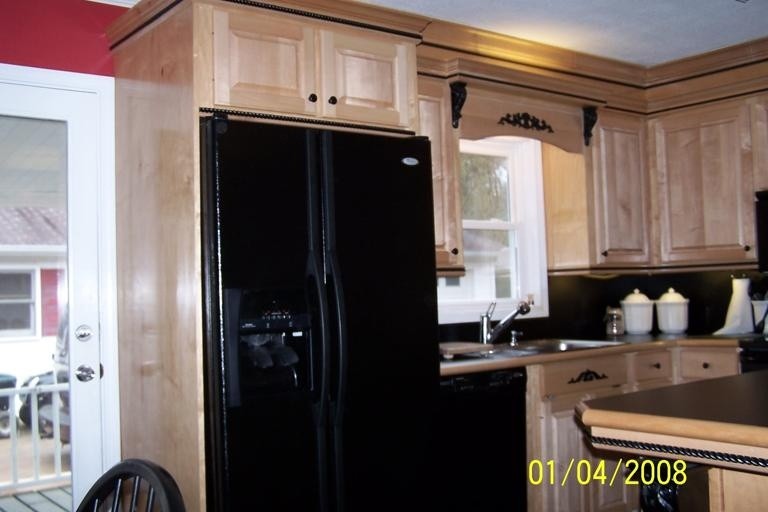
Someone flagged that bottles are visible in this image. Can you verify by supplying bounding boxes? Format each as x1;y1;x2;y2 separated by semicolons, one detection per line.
605;308;624;336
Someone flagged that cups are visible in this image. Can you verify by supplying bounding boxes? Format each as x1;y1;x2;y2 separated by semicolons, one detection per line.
618;286;692;336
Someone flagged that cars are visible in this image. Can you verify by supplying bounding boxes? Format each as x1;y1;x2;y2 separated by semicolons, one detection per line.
17;370;70;436
36;384;74;446
0;373;20;440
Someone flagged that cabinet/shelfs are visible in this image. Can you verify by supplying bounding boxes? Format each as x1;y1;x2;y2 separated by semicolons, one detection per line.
679;348;740;378
630;352;671;379
539;354;638;512
592;110;651;266
415;74;465;277
114;3;420;131
647;94;757;268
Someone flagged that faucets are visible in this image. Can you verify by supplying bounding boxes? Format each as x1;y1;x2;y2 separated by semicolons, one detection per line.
478;298;531;341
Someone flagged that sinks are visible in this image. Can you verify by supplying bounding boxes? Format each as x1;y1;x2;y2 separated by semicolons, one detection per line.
507;339;629;354
465;347;541;362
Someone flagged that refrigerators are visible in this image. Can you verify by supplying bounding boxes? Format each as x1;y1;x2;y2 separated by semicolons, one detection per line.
199;115;444;512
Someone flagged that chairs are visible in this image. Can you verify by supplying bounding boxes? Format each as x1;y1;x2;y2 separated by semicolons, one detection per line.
73;460;186;511
